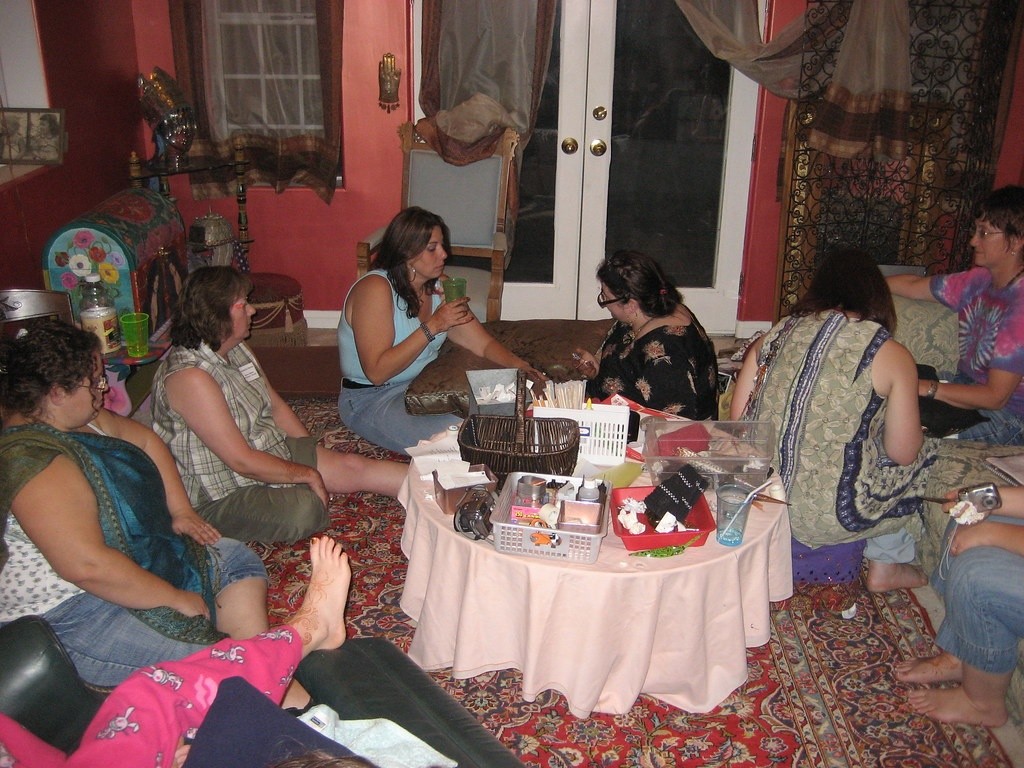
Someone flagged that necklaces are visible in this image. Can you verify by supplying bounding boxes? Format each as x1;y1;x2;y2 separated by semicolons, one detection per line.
634;316;651;337
1003;267;1024;287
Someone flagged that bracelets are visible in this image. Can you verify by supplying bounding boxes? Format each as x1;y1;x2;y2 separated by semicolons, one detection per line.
420;321;435;342
928;378;938;399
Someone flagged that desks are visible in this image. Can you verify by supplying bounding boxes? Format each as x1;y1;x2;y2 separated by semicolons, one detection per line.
398;403;793;717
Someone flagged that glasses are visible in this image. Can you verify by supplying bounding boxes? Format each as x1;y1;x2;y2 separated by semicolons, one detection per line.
597;289;623;309
75;377;106;392
970;224;1008;239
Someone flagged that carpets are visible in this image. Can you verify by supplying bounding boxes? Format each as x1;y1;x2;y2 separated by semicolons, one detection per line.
196;398;1009;767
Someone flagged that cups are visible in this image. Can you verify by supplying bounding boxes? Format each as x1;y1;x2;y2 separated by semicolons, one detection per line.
120;313;150;357
442;278;467;302
716;483;754;546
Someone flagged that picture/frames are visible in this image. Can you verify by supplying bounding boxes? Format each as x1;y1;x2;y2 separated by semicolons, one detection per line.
0;107;63;165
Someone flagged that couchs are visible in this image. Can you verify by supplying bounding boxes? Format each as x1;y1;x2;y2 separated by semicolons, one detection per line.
1;615;527;768
873;294;1024;737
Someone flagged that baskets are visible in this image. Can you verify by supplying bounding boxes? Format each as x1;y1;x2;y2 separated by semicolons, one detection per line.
532;399;631;467
458;368;581;489
489;472;612;565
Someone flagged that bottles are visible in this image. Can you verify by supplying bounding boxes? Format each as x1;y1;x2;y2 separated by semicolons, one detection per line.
80;274;121;358
579;478;599;503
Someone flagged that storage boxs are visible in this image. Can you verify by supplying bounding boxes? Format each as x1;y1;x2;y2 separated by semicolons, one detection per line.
610;486;716;550
643;418;776;493
433;463;497;515
489;472;613;565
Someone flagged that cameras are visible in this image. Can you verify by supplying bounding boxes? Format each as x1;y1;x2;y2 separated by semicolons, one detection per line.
959;483;1002;513
458;490;498;539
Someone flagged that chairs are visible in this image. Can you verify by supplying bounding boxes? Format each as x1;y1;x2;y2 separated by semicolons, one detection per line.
356;114;521;323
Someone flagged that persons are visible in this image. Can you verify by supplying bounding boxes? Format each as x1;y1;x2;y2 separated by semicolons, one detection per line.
573;248;720;421
148;265;411;547
1;315;351;768
895;488;1024;727
264;732;380;768
337;206;551;458
731;251;930;592
884;183;1024;447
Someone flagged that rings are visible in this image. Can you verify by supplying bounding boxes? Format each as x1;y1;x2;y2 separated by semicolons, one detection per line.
584;361;589;367
